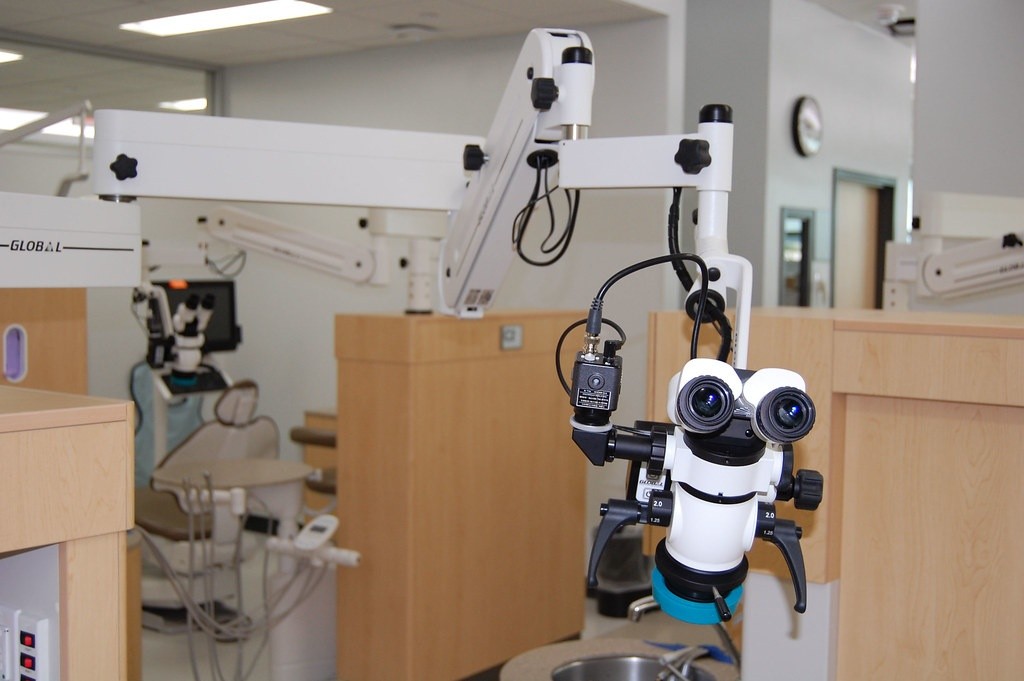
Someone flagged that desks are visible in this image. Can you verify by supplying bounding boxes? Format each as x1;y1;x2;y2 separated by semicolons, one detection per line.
152;458;315;681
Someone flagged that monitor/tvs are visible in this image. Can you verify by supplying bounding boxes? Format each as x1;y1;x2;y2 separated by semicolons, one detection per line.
149;279;242;352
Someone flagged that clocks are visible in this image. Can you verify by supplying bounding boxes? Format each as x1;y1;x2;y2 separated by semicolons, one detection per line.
794;95;823;155
